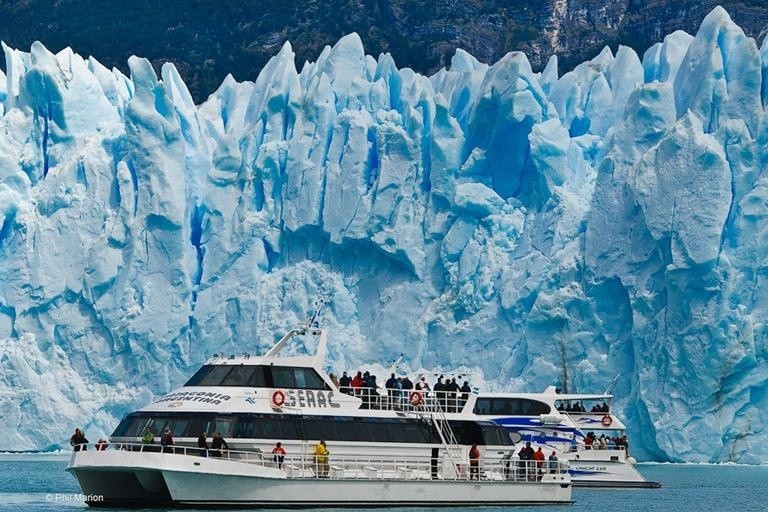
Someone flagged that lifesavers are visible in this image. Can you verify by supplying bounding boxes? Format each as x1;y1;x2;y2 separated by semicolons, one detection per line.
409;391;422;405
602;416;612;427
272;391;285;406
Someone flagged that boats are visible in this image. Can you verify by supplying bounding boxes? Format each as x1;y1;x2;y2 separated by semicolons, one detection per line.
64;291;580;506
475;373;663;488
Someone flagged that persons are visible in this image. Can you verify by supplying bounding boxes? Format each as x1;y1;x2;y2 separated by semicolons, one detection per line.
329;371;470;413
583;432;629;450
570;435;577;452
162;426;175;453
142;426;154;449
95;439;108;451
515;399;527;415
198;431;209;456
70;428;88;451
211;432;228;456
591;402;609;412
518;442;558;480
469;442;479;480
504;401;512;414
272;442;286;468
557;402;585;411
313;438;329;478
